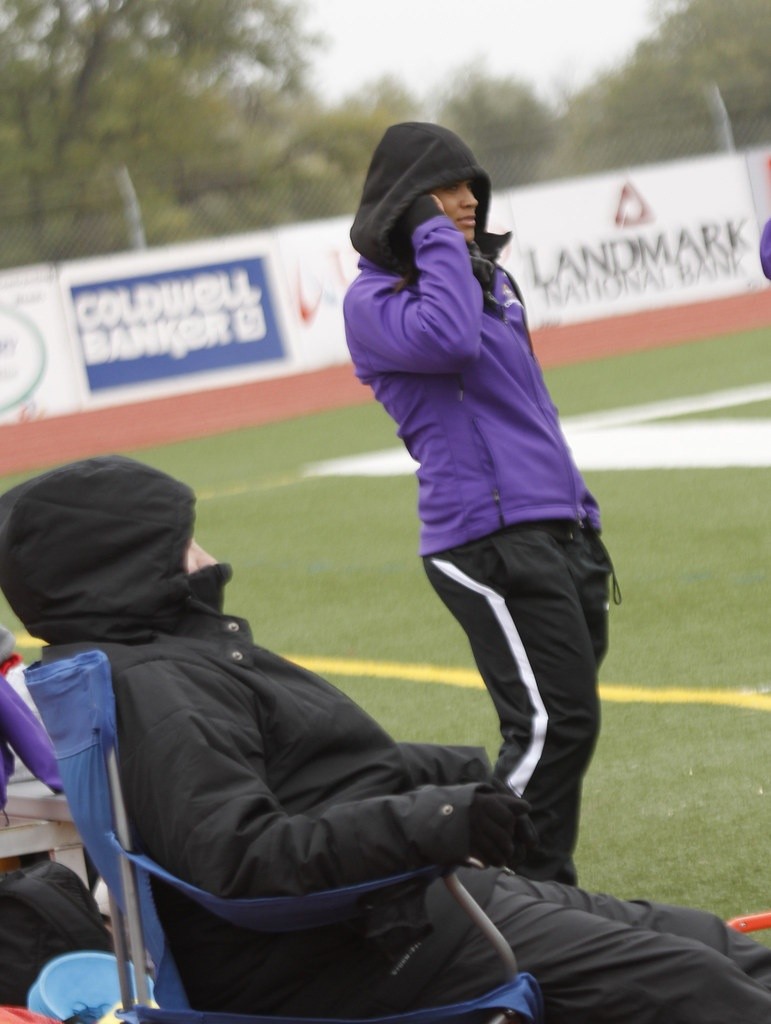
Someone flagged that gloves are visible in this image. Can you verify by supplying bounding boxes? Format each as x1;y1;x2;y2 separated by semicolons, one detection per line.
469;794;538;865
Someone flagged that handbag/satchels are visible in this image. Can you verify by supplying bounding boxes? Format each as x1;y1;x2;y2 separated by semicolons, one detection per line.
0;861;114;1007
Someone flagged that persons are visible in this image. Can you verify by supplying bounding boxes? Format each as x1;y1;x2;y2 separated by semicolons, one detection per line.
1;454;770;1024
342;121;625;883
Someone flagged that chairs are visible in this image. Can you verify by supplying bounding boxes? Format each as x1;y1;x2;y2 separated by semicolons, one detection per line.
21;650;540;1023
